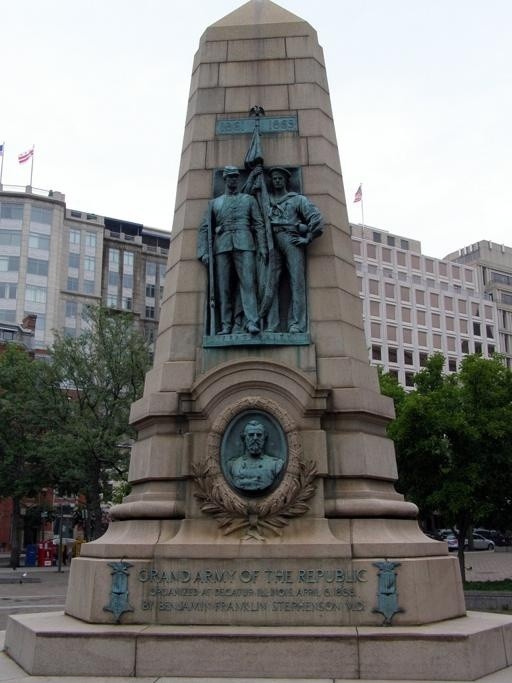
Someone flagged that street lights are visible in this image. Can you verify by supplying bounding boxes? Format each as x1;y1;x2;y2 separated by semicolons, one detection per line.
41;504;88;573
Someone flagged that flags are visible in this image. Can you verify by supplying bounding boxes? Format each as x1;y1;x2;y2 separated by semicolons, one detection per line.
18;147;33;164
0;144;3;156
354;185;362;203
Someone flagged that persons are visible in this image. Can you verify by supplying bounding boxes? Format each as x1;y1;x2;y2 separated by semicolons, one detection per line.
231;420;284;489
196;165;325;336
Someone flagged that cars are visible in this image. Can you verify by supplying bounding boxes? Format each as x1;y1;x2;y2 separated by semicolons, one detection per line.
425;528;512;551
16;536;86;566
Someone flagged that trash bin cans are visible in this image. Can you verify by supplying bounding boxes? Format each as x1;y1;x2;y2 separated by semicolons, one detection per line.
26;544;50;566
